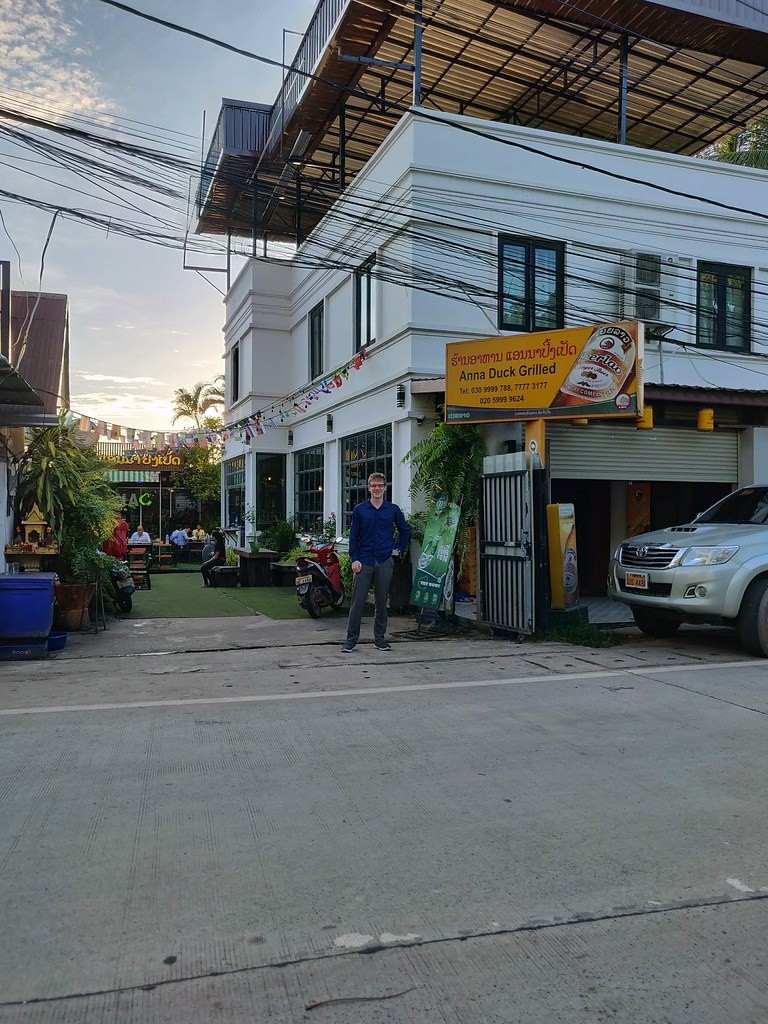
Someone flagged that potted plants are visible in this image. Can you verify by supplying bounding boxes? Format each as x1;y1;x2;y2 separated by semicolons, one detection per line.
54;534;126;632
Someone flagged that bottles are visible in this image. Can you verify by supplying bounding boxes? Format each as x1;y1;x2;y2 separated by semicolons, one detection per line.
165;534;169;545
417;523;447;568
546;322;640;409
195;532;199;540
562;522;579;608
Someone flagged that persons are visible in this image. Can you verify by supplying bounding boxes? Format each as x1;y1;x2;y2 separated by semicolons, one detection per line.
192;523;204;537
169;524;193;564
131;526;151;565
340;473;410;653
200;531;227;588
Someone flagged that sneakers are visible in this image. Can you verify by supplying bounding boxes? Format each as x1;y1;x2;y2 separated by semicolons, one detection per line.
374;640;391;650
340;642;356;652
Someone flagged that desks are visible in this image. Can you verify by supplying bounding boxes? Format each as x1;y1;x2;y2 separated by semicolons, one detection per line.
123;539;153;568
155;543;172;569
233;547;278;587
188;540;206;565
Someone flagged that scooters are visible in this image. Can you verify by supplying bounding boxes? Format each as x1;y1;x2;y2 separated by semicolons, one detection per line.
110;560;135;614
290;535;345;618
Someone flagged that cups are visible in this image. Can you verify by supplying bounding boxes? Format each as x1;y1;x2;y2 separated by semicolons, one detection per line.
12;562;19;574
4;563;12;576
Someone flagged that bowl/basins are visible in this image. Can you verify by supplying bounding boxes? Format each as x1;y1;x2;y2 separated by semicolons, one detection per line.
48;630;70;650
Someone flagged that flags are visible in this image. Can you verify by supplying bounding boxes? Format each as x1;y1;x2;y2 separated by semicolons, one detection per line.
58;349;369;460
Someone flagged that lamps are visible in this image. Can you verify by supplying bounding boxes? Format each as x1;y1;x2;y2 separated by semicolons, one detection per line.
397;384;405;407
326;413;332;432
288;430;293;445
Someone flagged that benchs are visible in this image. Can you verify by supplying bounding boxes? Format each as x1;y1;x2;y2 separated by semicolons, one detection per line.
205;565;240;587
270;562;299;587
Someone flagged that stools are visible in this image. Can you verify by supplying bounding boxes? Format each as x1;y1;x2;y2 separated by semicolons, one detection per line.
155;554;171;560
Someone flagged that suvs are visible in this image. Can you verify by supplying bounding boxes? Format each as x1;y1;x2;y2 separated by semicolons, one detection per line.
605;485;768;658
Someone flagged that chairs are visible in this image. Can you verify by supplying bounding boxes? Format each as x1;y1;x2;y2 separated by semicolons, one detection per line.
172;539;190;568
129;553;152;590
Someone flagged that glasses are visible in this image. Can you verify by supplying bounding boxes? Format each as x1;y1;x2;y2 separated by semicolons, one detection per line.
371;484;384;487
